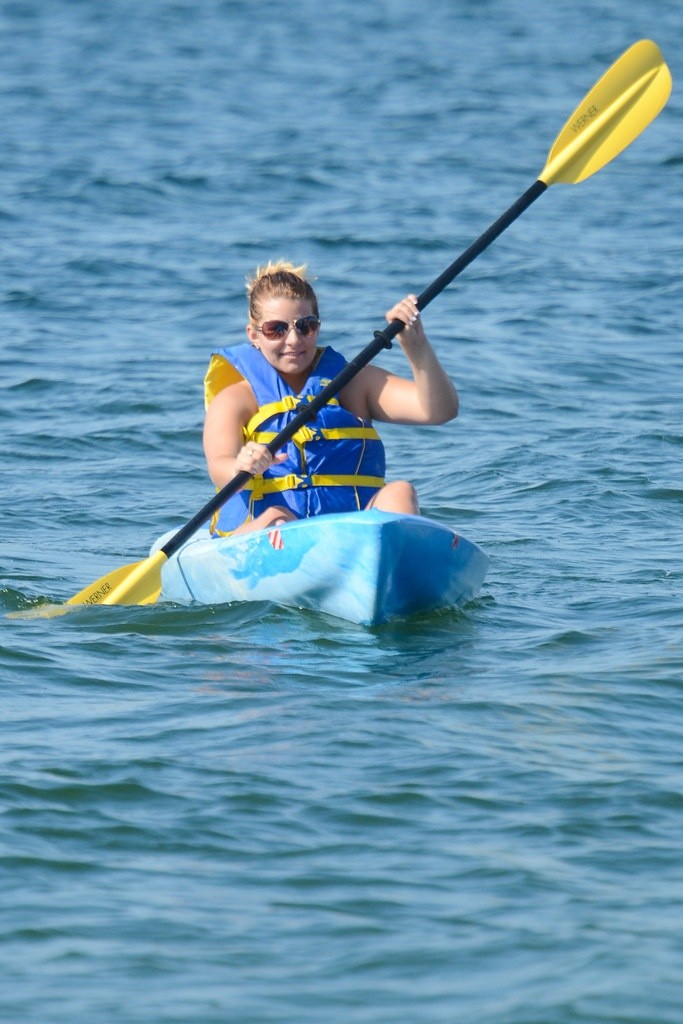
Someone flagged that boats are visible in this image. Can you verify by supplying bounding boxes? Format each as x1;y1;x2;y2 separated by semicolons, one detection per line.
149;509;493;633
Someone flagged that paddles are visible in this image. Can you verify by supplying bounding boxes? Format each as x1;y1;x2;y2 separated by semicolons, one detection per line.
65;35;674;608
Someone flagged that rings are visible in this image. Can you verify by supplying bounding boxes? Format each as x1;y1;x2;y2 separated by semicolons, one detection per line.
248;450;254;457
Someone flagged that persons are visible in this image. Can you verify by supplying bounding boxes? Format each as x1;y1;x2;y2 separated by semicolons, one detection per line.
203;263;460;537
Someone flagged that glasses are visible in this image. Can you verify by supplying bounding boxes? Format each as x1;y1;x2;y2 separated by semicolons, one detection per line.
253;314;320;340
